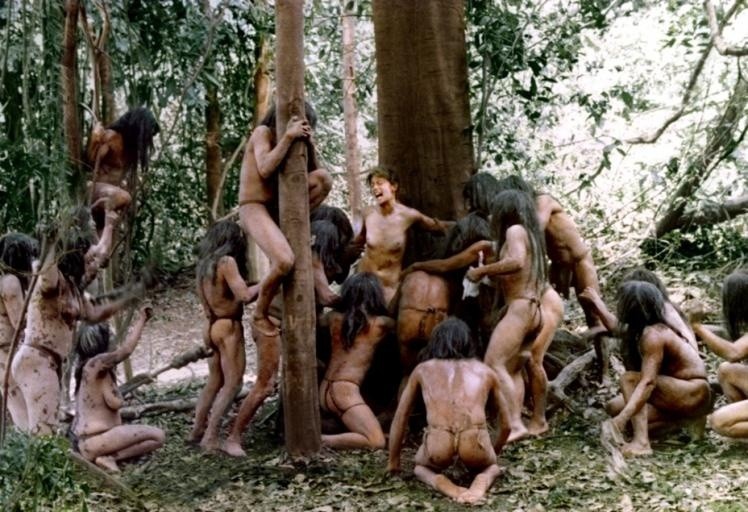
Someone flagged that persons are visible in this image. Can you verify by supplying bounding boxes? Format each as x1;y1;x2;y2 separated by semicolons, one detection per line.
0;102;748;476
389;316;511;505
